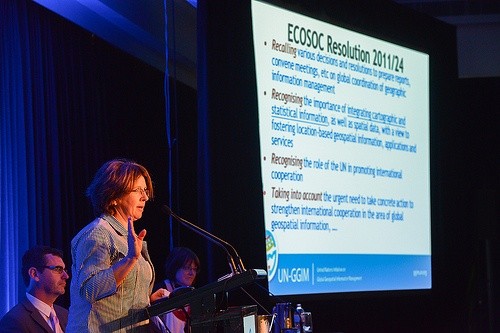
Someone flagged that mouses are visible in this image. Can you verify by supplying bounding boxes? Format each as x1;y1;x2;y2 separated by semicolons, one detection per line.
169;286;195;299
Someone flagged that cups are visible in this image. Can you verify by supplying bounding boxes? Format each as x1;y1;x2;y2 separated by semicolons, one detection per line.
301;312;313;333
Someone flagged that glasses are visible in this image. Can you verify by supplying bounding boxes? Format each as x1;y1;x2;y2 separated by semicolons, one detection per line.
35;266;67;274
182;266;198;273
130;188;150;196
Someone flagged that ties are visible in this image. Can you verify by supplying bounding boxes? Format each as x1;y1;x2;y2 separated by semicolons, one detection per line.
50;313;56;333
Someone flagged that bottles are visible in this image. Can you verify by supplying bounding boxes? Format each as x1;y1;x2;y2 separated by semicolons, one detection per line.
294;304;304;333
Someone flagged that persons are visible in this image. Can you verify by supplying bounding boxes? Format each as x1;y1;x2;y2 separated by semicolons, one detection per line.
65;160;153;333
152;248;199;333
0;244;69;333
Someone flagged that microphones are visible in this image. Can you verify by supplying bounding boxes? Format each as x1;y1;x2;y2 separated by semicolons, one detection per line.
161;205;247;277
